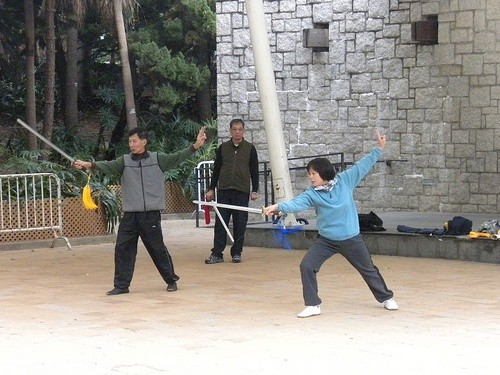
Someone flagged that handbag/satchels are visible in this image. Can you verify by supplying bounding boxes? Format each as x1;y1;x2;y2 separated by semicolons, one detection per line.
478;219;500;234
444;216;472;236
359;211;385;232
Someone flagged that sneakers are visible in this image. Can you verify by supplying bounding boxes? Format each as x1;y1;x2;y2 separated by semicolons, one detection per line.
106;287;129;295
232;253;241;263
167;281;177;291
205;253;224;264
297;305;322;318
384;300;398;310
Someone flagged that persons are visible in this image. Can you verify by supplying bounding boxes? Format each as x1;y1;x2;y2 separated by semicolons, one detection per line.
73;125;207;294
265;130;399;318
204;119;260;265
